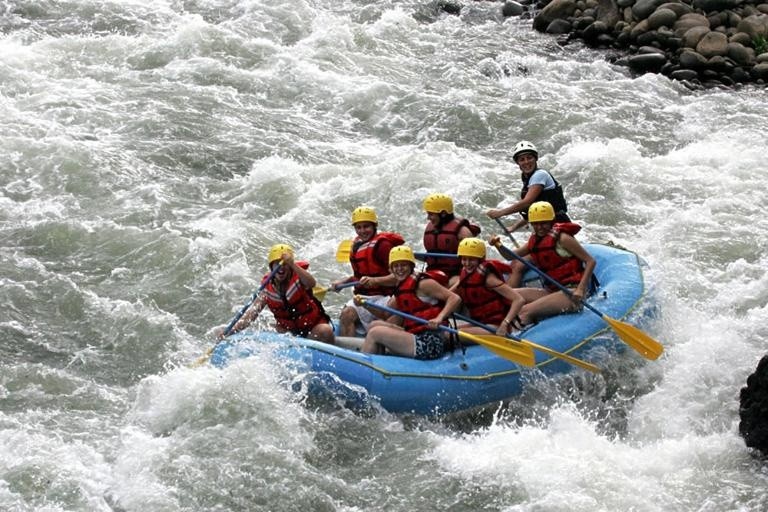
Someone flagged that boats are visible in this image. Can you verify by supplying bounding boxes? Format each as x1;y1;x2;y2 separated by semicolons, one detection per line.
210;244;662;427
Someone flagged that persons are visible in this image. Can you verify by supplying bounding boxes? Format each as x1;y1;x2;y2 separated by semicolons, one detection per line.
220;244;334;343
411;194;473;290
447;238;524;346
330;206;397;340
488;139;571;234
354;246;461;359
488;201;595;326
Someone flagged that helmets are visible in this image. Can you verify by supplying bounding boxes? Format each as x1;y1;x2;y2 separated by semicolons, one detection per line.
270;245;294;267
352;207;379;226
459;238;488;258
513;141;540;160
423;194;453;214
528;201;556;222
390;246;416;266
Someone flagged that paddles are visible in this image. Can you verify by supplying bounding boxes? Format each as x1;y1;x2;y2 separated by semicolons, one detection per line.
335;240;460;263
189;254;293;369
313;280;367;302
491;235;664;360
452;312;601;373
355;295;535;366
